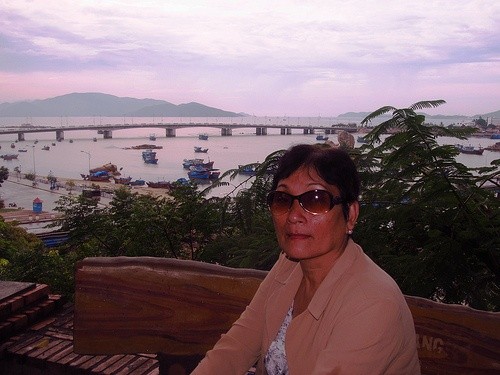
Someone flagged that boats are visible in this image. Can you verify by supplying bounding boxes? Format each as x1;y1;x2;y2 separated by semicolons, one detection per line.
238;160;262;176
146;180;171;188
316;133;329;141
80;160;146;187
182;155;221;184
490;134;500;139
198;133;209;140
142;149;159;164
193;146;209;153
454;142;500;155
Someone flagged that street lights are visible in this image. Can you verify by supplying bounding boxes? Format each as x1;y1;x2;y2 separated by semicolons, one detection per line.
81;151;91;190
32;145;36;175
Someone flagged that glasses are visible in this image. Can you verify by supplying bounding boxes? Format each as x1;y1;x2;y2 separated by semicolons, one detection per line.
267;189;343;216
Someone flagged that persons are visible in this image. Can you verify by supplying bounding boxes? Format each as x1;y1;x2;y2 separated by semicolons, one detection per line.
191;144;421;375
